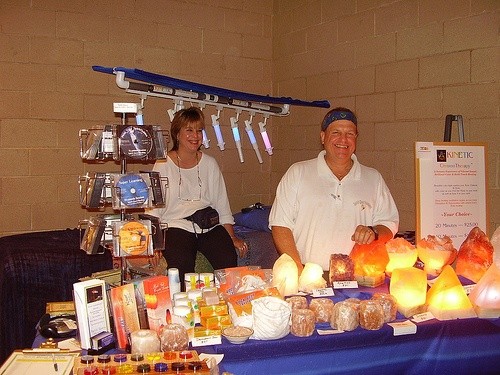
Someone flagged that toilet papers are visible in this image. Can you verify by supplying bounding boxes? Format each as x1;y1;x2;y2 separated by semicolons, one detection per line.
128;328;158;353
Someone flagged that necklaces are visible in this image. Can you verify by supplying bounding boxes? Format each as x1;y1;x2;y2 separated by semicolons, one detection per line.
175;150;202;202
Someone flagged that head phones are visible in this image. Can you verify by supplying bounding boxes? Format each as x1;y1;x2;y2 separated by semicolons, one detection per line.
36;313;78;339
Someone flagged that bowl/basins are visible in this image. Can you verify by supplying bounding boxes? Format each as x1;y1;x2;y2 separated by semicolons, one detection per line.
222;327;254;344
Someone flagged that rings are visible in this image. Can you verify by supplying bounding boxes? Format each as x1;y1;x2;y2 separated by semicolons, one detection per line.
239;248;243;250
243;245;246;249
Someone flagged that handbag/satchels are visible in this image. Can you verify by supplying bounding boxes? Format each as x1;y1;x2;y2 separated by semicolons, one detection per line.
183;206;220;238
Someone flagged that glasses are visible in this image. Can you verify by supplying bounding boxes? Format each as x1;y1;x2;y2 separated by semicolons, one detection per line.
178;183;202;202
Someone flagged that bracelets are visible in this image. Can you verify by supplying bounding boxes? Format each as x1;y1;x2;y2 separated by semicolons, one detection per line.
368;225;378;240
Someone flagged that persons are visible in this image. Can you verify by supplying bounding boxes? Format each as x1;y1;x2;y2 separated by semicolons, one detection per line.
144;106;248;292
269;106;399;277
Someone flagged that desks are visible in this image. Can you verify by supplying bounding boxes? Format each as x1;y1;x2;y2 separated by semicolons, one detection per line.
32;266;500;375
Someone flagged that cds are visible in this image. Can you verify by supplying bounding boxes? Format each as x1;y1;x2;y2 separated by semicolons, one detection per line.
119;126;153;159
116;175;148;207
119;221;150;255
